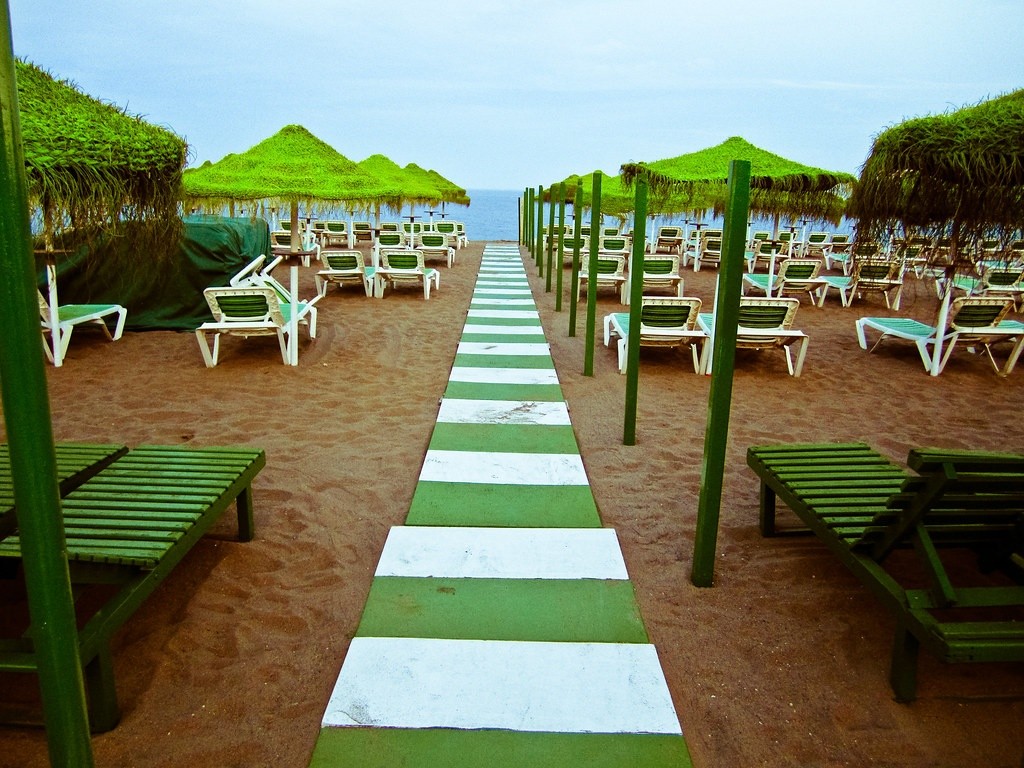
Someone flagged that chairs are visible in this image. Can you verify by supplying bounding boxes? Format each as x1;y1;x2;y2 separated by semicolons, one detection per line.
0;218;468;768
531;225;1024;768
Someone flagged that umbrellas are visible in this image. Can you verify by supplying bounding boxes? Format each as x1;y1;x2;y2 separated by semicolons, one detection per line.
181;124;470;367
855;90;1024;266
535;136;858;373
14;58;188;264
